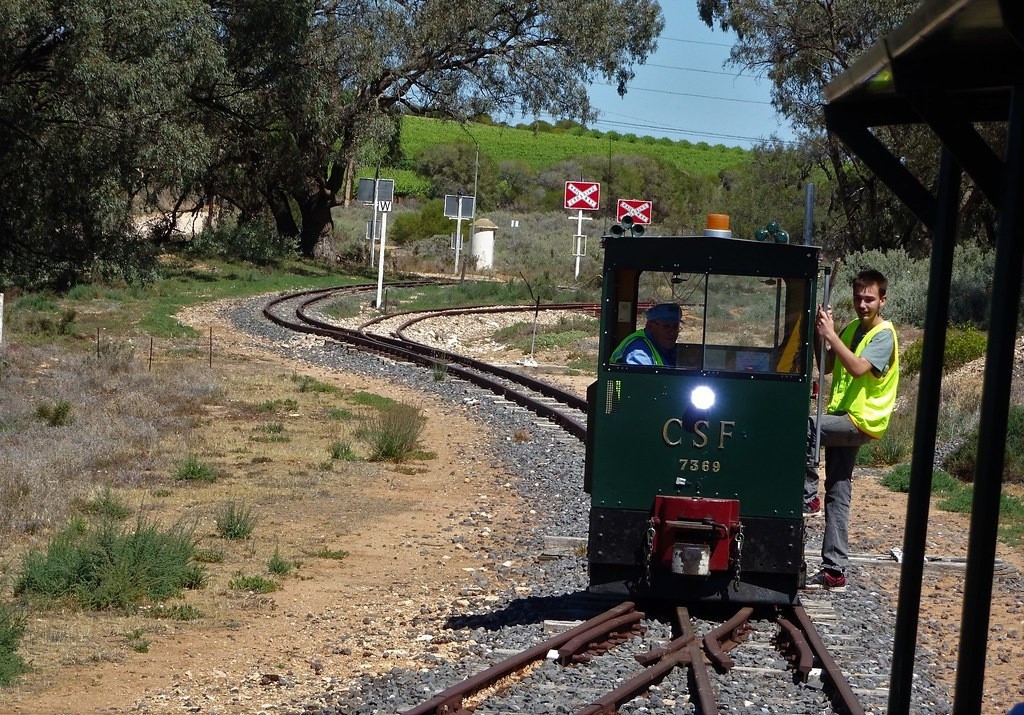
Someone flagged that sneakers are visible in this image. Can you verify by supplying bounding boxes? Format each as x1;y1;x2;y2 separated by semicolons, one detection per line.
802;497;821;517
805;569;845;593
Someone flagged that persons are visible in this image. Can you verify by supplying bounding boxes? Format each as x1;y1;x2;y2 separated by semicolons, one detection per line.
610;303;684;370
805;268;900;590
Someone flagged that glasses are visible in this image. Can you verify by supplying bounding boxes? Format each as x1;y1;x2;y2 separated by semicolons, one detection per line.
655;320;683;332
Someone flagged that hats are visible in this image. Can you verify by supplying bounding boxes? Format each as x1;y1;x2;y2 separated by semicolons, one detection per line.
646;303;686;324
